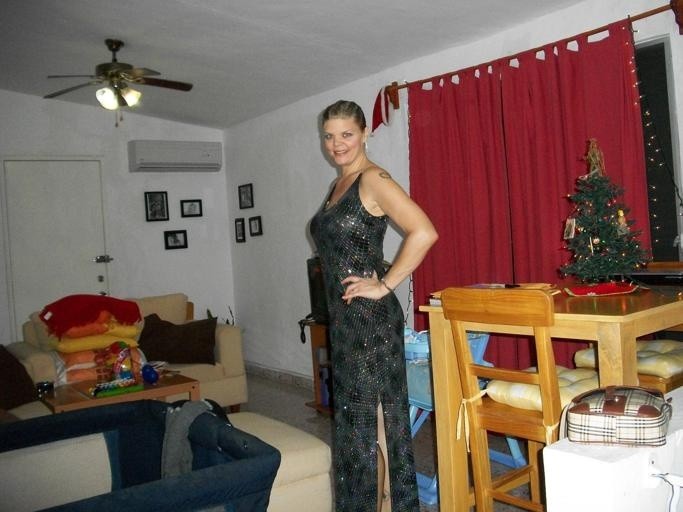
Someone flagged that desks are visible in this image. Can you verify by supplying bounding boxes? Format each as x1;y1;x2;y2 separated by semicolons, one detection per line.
300;317;332;415
418;280;683;512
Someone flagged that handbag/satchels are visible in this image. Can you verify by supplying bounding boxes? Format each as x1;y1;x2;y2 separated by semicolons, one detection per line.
559;385;672;446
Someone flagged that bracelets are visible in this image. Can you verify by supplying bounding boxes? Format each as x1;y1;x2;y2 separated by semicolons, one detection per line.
382;279;394;293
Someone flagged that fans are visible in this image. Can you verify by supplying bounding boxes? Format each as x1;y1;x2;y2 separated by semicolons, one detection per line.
43;38;193;98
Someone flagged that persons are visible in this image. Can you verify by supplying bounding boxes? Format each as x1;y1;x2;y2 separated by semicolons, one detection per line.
310;101;439;506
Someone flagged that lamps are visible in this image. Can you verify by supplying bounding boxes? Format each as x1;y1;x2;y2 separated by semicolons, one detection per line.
95;79;142;110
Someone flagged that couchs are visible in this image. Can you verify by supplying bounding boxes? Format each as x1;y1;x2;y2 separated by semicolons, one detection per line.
0;293;249;414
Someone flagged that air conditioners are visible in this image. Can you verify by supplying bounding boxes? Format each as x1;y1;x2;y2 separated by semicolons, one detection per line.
127;140;222;172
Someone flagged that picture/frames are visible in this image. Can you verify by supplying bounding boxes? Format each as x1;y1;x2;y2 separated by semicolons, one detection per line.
145;191;203;250
235;183;263;243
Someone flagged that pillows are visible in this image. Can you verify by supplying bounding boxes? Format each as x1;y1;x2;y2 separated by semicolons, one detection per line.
28;292;146;388
138;313;217;366
0;344;38;415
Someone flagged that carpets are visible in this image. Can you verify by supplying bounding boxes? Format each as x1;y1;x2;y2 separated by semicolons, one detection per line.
307;401;333;417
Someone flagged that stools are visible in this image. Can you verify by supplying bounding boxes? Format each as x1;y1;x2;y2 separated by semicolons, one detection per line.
225;410;333;512
573;337;683;394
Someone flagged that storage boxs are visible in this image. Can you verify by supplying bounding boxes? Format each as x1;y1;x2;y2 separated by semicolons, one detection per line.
0;398;282;512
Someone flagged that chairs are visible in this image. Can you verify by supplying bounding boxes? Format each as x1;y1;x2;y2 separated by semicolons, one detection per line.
437;288;643;512
399;328;529;504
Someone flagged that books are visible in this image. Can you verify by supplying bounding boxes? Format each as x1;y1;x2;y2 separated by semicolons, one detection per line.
429;282;562;307
